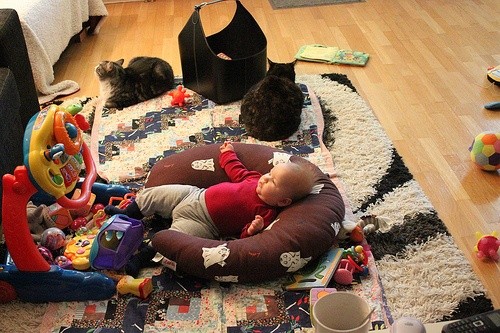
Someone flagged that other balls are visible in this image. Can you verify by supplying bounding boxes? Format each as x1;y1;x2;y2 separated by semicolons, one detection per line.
469;131;500;171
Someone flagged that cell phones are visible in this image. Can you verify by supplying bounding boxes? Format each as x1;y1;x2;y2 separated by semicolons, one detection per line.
441;308;500;333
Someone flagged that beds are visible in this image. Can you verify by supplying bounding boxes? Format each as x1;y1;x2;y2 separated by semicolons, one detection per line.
0;0;108;105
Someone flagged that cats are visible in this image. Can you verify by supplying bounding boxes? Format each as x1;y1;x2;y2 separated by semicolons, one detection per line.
94;56;174;117
239;58;305;142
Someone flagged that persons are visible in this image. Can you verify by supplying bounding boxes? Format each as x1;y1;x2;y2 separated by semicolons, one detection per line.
104;141;314;279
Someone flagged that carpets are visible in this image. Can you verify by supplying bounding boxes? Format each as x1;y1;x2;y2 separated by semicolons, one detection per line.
0;70;496;333
